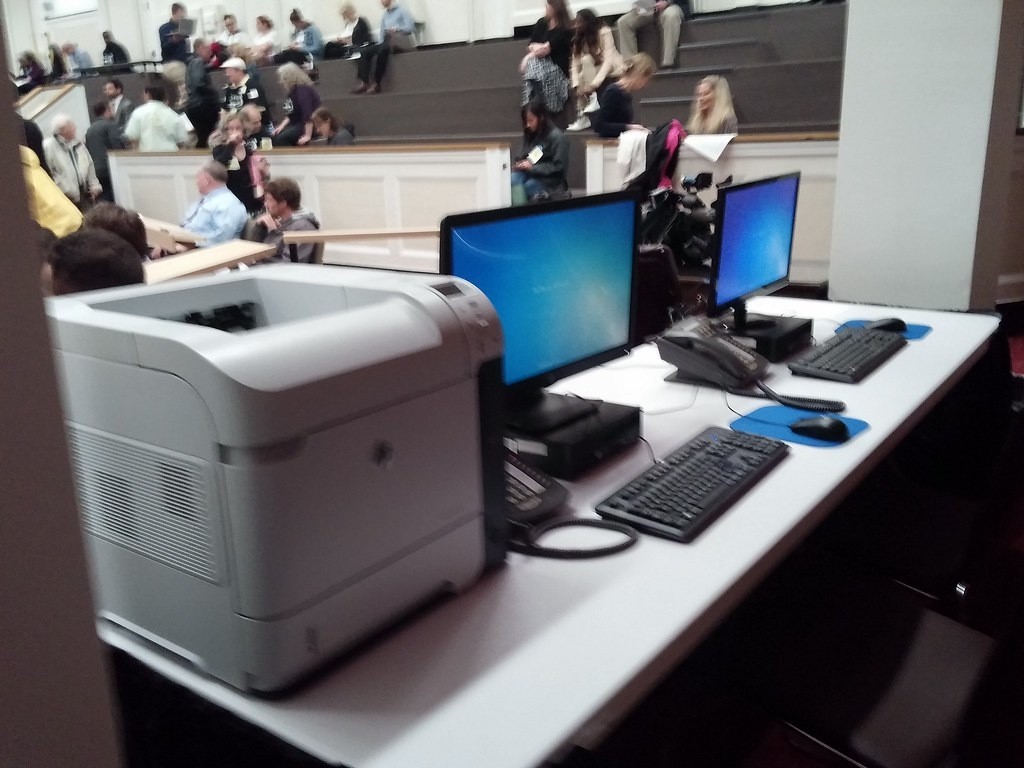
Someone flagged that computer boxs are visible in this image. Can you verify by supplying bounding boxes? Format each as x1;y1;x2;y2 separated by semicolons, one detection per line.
717;313;813;363
502;391;641;481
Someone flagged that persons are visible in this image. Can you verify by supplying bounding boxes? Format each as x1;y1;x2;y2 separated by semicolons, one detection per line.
617;0;694;71
8;0;415;295
567;8;626;134
685;74;739;136
512;98;571;204
590;52;657;137
526;0;575;132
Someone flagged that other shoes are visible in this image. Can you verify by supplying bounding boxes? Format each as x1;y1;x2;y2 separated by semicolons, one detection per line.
365;82;382;95
351;81;370;94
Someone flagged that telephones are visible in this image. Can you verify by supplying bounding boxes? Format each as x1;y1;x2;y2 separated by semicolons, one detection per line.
506;445;570;534
655;317;770;390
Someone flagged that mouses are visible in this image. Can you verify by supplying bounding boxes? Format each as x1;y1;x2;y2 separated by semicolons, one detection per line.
866;317;907;332
789;416;850;442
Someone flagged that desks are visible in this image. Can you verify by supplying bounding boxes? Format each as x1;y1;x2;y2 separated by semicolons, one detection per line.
98;293;1024;767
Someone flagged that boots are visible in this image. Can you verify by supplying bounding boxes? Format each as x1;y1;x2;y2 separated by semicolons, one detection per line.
583;92;601;116
566;110;592;132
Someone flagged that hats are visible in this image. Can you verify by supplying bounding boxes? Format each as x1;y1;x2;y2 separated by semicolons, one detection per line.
218;57;247;70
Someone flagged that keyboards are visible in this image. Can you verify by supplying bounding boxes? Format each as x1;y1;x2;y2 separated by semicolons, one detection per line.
788;328;907;384
595;426;788;544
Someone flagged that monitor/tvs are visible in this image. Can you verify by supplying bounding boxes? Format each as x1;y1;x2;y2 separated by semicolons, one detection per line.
439;188;642;435
707;170;800;332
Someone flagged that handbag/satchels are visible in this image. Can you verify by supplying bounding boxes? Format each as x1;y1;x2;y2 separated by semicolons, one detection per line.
390;31;417;54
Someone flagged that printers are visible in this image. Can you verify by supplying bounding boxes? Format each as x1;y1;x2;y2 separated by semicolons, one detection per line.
44;260;508;699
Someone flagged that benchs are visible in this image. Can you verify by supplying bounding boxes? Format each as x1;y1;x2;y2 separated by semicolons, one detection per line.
116;0;839;147
